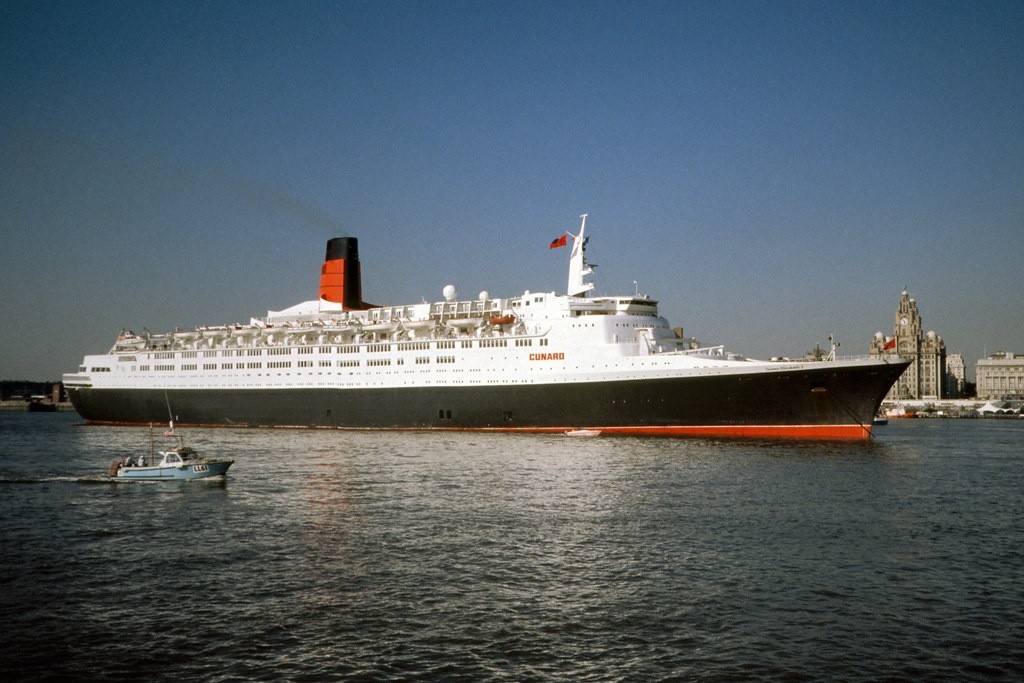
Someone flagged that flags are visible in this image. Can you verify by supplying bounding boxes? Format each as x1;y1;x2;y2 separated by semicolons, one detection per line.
884;339;895;350
550;236;567;248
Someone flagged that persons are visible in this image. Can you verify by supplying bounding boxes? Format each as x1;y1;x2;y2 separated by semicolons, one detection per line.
138;454;148;466
125;454;135;467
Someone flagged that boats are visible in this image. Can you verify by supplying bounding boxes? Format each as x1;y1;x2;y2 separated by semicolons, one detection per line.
110;391;234;487
873;411;888;425
878;411;915;418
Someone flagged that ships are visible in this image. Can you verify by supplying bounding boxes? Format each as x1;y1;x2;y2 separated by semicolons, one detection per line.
59;209;917;443
